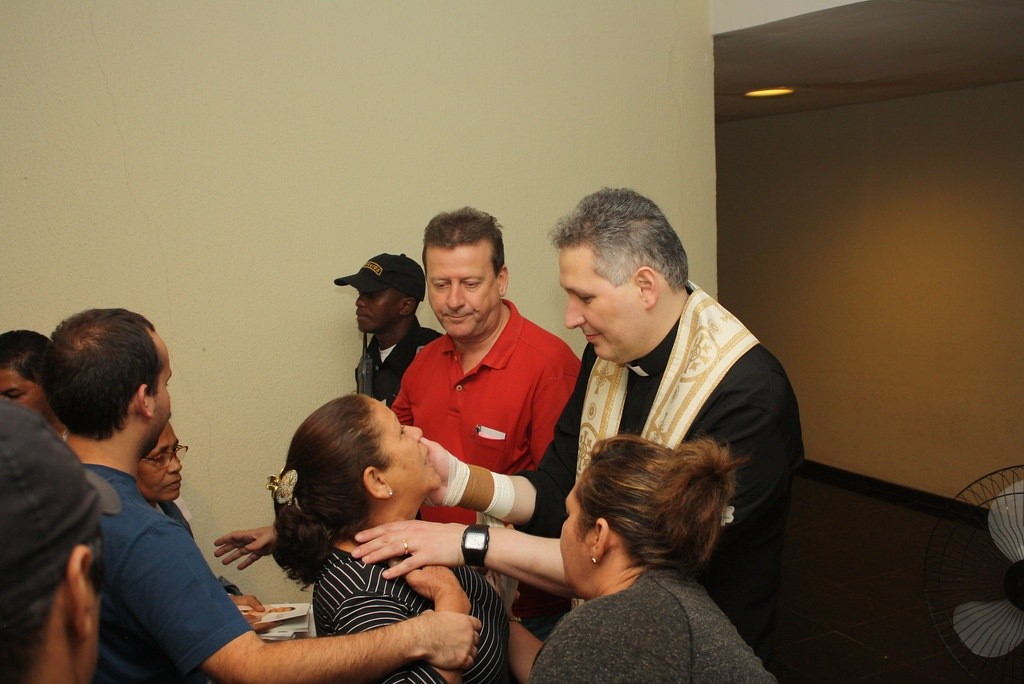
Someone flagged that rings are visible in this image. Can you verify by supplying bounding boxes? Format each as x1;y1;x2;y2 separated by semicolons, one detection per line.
403;539;408;554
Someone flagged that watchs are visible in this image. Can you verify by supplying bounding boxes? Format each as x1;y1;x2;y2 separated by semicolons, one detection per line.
461;523;491;568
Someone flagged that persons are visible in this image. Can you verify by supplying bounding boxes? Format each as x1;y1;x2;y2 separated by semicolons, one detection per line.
48;307;482;684
267;396;514;684
214;205;580;645
0;402;105;684
352;186;805;662
0;330;67;442
240;605;295;618
137;423;275;635
507;435;779;684
334;253;444;411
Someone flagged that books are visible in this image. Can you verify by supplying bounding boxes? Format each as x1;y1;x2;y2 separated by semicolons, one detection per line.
237;603;317;641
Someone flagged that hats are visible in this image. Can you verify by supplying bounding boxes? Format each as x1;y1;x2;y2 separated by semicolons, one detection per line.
0;399;102;634
334;253;426;301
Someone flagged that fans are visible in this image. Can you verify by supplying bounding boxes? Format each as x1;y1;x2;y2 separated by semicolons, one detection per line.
922;464;1024;684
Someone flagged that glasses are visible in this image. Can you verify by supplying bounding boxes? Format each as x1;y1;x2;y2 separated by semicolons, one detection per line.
140;445;188;469
